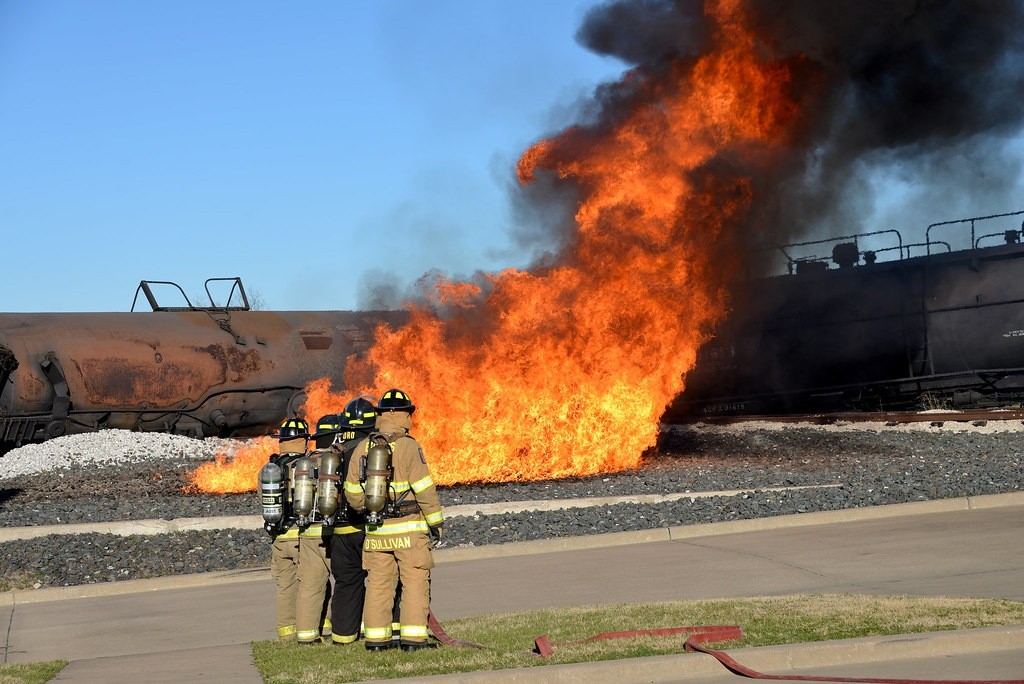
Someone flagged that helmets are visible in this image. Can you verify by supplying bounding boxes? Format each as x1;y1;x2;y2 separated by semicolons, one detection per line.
338;397;377;428
373;389;416;416
271;418;311;440
310;414;341;441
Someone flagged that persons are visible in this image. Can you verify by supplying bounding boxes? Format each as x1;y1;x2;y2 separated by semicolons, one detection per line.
257;389;444;651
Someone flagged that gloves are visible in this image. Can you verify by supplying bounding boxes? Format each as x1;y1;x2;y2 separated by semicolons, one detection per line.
427;525;443;550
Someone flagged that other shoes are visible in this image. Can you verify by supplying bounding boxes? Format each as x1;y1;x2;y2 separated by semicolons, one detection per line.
300;635;326;645
365;640;398;652
401;642;441;652
